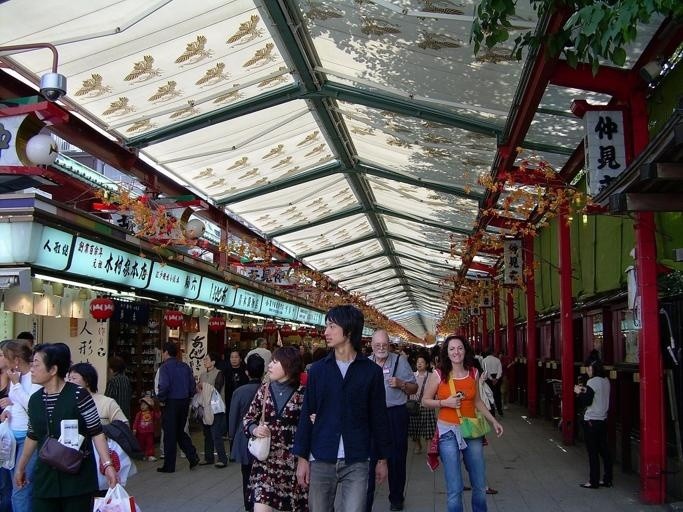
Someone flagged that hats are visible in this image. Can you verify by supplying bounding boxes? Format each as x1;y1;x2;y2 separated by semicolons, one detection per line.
138;396;155;408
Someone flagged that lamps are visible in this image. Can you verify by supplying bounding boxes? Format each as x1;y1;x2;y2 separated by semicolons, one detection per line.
184;219;205;240
25;134;59;169
79;287;91;301
31;278;43;295
52;282;64;298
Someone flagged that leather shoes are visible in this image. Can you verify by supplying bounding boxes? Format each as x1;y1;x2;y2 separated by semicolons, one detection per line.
388;499;405;512
463;484;471;493
156;465;176;473
188;455;201;471
597;478;614;489
579;481;599;489
486;486;498;496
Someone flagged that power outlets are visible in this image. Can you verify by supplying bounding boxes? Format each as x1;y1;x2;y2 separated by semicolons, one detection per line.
115;307;164;409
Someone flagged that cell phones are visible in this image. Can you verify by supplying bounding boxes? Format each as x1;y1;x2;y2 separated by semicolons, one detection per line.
456;392;464;400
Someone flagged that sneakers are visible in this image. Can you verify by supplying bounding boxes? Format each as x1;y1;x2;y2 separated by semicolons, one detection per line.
491;409;504;417
412;445;422;455
214;459;229;468
197;457;215;467
230;457;237;464
142;450;187;461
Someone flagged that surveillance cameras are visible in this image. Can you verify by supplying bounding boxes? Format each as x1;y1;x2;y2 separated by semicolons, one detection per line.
40;72;67;102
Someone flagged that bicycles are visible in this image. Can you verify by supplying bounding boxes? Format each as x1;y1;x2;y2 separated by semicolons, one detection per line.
544;375;584;432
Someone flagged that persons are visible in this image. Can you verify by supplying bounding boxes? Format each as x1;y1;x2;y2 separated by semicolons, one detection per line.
290;302;394;511
0;338;45;512
365;327;419;510
229;352;266;511
12;342;121;512
462;347;504;493
398;345;442;456
572;359;614;489
2;331;131;494
0;418;12;512
419;333;505;511
106;338;327;472
241;344;318;511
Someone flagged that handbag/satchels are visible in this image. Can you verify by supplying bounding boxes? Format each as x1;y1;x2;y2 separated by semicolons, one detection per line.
456;411;492;440
0;416;18;471
405;399;422;417
583;419;606;429
38;435;86;478
246;422;272;462
91;434;139;492
